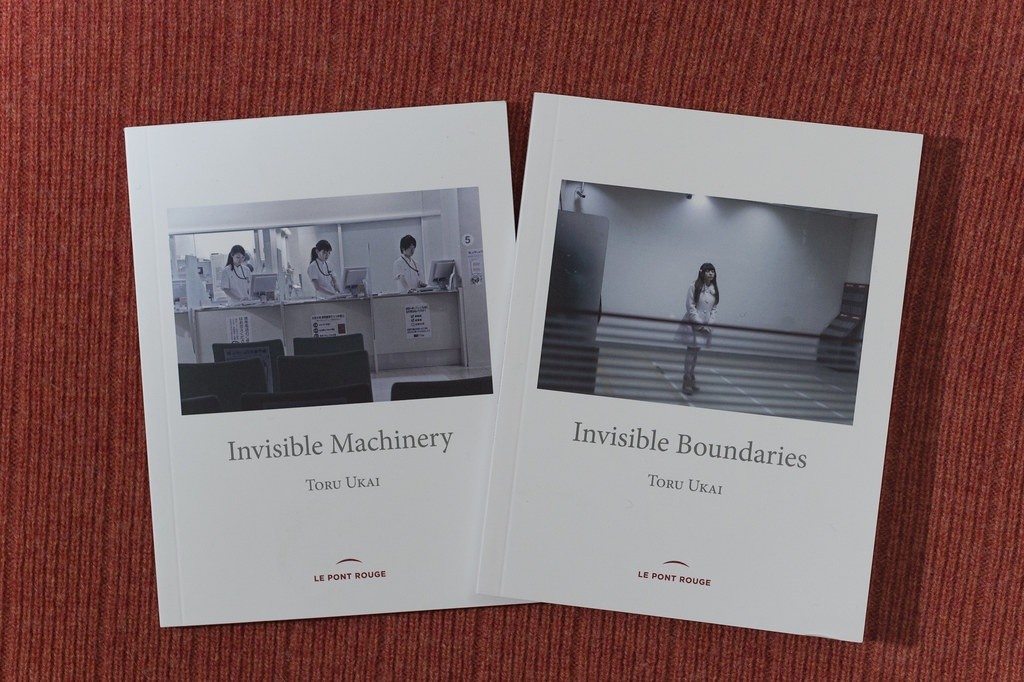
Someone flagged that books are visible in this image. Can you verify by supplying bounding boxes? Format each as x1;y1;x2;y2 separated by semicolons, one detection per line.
475;92;927;641
122;100;545;626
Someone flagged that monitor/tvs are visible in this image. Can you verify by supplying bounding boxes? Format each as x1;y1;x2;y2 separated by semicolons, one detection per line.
342;266;368;298
428;258;456;292
172;279;187;307
250;273;277;304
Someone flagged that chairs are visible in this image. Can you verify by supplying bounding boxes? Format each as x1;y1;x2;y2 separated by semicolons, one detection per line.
178;333;494;415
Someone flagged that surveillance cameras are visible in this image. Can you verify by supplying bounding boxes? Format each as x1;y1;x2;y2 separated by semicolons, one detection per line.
576;191;586;198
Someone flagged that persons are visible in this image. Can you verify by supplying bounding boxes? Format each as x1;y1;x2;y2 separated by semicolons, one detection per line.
394;234;427;294
220;245;253;305
676;262;720;395
307;240;341;298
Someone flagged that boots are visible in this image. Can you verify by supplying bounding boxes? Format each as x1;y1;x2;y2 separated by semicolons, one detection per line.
683;376;700;394
